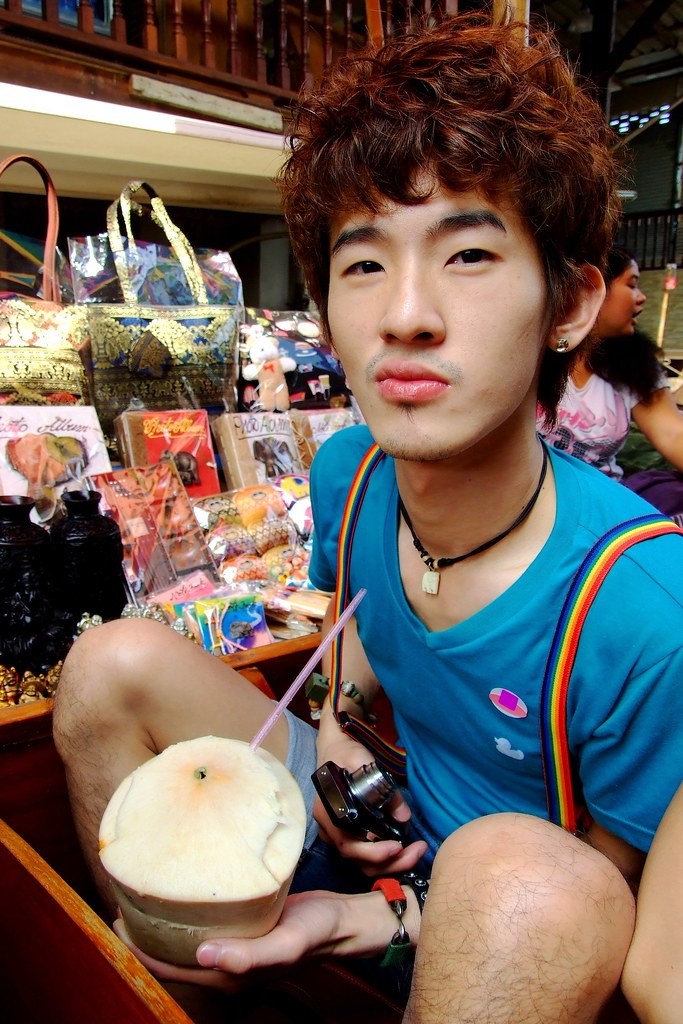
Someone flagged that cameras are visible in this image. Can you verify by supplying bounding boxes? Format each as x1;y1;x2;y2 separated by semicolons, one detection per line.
310;760;404;846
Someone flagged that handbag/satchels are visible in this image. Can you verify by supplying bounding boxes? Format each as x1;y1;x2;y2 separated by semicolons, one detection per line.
0;153;91;406
84;181;241;460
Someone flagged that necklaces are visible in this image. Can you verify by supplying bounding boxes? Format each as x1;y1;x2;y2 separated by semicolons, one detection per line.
398;438;548;596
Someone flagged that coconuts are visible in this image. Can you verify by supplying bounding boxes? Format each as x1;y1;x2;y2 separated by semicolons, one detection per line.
98;737;305;966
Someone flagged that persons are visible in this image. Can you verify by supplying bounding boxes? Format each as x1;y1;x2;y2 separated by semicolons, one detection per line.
618;783;683;1024
52;13;683;1024
535;247;683;532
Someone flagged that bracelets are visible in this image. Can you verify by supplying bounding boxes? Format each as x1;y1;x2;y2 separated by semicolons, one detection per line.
370;878;414;969
305;671;380;732
399;873;430;916
